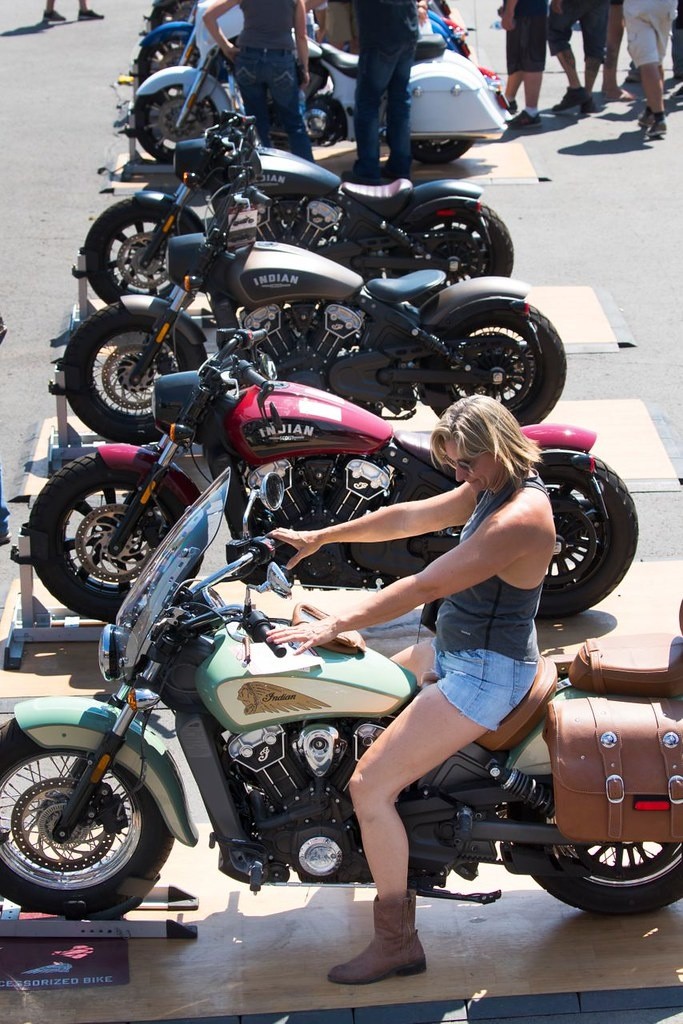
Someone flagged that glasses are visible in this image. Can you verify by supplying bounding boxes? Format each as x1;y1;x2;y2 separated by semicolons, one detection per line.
443;453;480;475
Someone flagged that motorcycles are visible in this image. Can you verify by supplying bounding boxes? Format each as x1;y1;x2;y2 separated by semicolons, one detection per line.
28;0;644;624
0;457;683;919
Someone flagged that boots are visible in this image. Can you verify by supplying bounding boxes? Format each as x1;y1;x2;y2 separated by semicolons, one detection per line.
327;889;426;985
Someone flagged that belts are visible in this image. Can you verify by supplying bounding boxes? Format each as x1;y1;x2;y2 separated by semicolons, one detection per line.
237;45;292;57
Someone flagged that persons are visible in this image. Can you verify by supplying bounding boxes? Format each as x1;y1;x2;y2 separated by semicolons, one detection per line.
192;0;682;186
42;0;104;22
264;394;557;984
0;314;12;546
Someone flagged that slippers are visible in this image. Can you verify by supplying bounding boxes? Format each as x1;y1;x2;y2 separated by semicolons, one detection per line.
603;89;635;102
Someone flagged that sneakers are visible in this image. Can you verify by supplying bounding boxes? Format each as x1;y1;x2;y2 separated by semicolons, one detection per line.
507;100;517;114
507;110;542;128
638;111;654;126
581;98;593;114
552;87;589;112
647;123;666;137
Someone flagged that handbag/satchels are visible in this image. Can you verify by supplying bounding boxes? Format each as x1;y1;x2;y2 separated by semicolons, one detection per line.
543;696;683;842
420;597;443;634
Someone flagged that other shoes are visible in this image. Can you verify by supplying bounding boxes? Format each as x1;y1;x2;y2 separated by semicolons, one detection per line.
674;66;683;78
676;87;683;98
0;532;12;543
378;165;394;181
43;10;65;21
79;10;104;19
629;69;642;81
341;170;379;187
